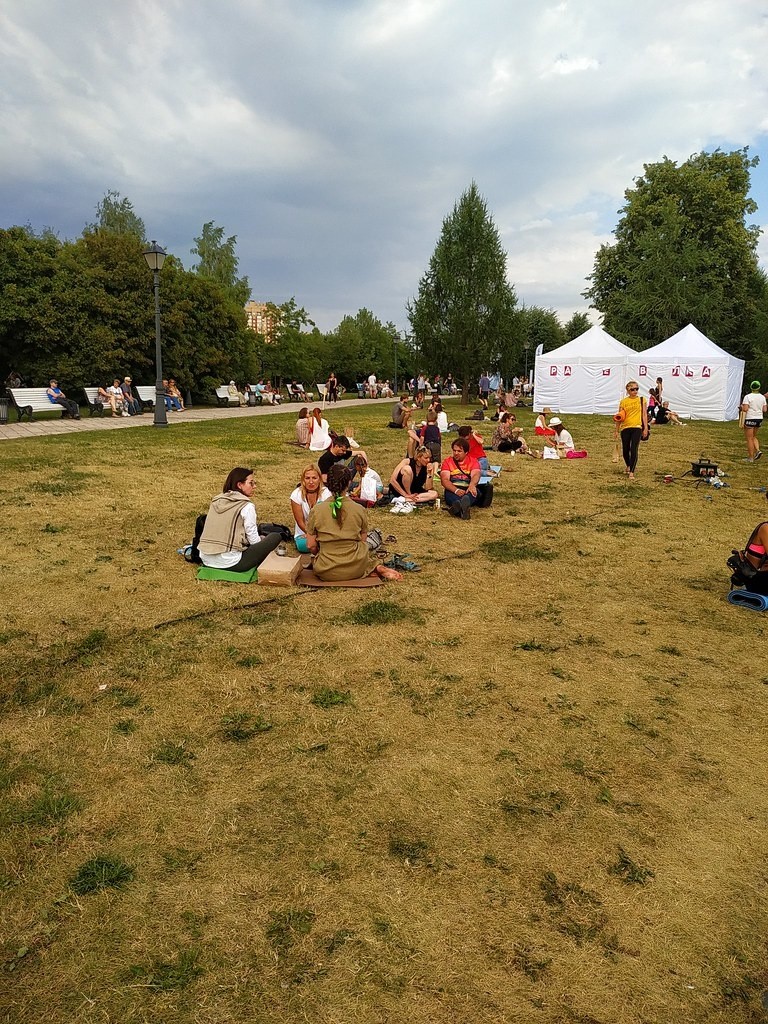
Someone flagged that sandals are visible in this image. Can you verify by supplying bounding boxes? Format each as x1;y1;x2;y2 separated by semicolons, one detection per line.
383;552;422;574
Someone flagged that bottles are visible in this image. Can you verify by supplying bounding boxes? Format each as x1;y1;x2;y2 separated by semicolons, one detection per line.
436;496;440;509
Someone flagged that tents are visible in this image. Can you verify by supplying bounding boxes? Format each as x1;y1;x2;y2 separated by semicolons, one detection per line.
533;325;639;416
626;323;746;422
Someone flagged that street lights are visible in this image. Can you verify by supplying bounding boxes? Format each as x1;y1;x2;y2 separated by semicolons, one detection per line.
393;336;400;397
142;240;168;427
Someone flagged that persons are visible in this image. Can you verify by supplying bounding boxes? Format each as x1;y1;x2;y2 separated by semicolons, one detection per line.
228;380;249;407
393;372;456;449
291;379;311;402
325;372;346;406
163;379;187;412
295;407;338;451
525;407;575;459
46;379;81;420
614;381;647;480
491;405;526;453
647;377;683;425
196;467;281;573
741;381;768;462
440;426;494;518
475;370;533;407
98;377;145;417
728;521;768;595
256;379;280;406
290;435;402;581
362;372;394;399
389;446;437;505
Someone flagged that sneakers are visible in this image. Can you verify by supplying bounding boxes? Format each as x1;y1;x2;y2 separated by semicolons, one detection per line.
390;501;413;513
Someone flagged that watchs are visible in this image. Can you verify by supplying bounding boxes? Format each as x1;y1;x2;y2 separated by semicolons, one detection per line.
427;474;432;478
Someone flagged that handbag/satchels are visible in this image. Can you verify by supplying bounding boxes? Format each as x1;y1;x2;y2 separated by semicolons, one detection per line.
739;410;746;428
543;446;560;460
256;550;304;587
329;430;338;441
612;443;620;462
640;424;650;440
475;482;493;508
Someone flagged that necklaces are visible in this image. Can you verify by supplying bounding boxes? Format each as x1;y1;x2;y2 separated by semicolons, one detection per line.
416;463;422;467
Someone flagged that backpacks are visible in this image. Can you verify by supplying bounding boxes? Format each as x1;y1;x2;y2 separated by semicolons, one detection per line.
183;514;208;564
427;425;441;463
258;522;294;543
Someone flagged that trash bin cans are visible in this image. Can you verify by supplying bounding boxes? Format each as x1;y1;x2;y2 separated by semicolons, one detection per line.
358;390;363;399
0;398;11;424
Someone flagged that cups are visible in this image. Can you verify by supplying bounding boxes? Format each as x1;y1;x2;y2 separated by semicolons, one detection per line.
665;475;672;484
511;451;515;456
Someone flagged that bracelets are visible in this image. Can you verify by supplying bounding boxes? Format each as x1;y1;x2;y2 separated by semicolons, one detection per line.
454;489;459;494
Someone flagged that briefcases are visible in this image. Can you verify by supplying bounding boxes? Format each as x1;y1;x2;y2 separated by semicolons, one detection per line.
691;459;718;477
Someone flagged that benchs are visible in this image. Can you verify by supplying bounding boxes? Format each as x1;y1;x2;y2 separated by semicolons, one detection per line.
406;383;437;396
285;384;315;402
452;383;462;393
83;387;129;416
316;384;342;400
214;385;240;408
135;386;175;412
5;388;79;422
249;385;281;406
356;383;394;398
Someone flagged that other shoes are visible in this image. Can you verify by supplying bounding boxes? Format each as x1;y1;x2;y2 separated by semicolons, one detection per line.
675;422;687;426
742;457;753;463
526;447;532;455
459;495;470;519
515;447;523;452
754;451;763;460
623;468;635;479
448;500;460;517
491;472;498;477
482;404;488;410
122;411;131;417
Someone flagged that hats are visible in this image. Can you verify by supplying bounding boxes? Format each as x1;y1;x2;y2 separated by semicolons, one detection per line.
548;417;562;426
541;407;551;413
750;381;761;390
124;377;132;381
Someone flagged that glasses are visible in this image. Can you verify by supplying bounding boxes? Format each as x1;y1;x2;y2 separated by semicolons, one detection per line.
630;388;638;391
512;418;516;421
242;481;257;486
